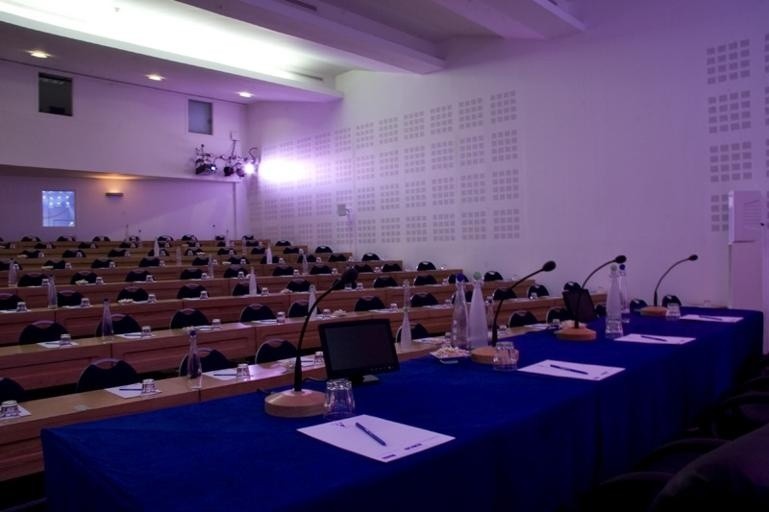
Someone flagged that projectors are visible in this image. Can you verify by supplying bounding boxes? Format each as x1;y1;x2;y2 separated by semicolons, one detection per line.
195;163;217;176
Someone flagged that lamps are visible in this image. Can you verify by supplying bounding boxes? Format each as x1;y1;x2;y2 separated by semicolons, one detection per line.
232;154;256;178
195;153;218;179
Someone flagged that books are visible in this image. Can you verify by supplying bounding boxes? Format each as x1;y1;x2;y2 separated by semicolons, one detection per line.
615;333;695;345
680;314;744;324
297;414;455;463
516;359;626;381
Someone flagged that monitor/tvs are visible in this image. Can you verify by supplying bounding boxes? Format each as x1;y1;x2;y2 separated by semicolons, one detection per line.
317;318;400;388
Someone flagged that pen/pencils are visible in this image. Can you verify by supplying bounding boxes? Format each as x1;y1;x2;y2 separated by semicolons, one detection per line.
120;389;143;390
260;321;276;322
47;343;61;344
534;325;548;328
214;374;238;376
551;365;587;374
315;315;330;318
422;339;438;341
301;360;314;362
642;336;667;341
700;315;722;320
125;334;143;336
356;423;388;445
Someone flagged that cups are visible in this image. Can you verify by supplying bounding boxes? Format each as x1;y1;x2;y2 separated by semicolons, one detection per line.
311;351;325;367
498;325;510;337
443;332;450;344
344;253;539;312
7;224;340;347
487;340;518;372
665;302;682;322
0;400;20;420
234;363;251;383
138;378;160;396
551;319;561;330
321;377;356;423
604;317;624;340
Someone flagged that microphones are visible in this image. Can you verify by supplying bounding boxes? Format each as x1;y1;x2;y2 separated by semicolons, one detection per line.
292;267;359;392
574;254;627;329
491;260;556;345
654;254;698;307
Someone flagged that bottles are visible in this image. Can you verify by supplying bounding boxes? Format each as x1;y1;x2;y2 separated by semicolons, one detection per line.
450;273;469;350
468;271;489;350
617;263;630;323
398;306;413;349
606;263;622;322
187;330;204;389
